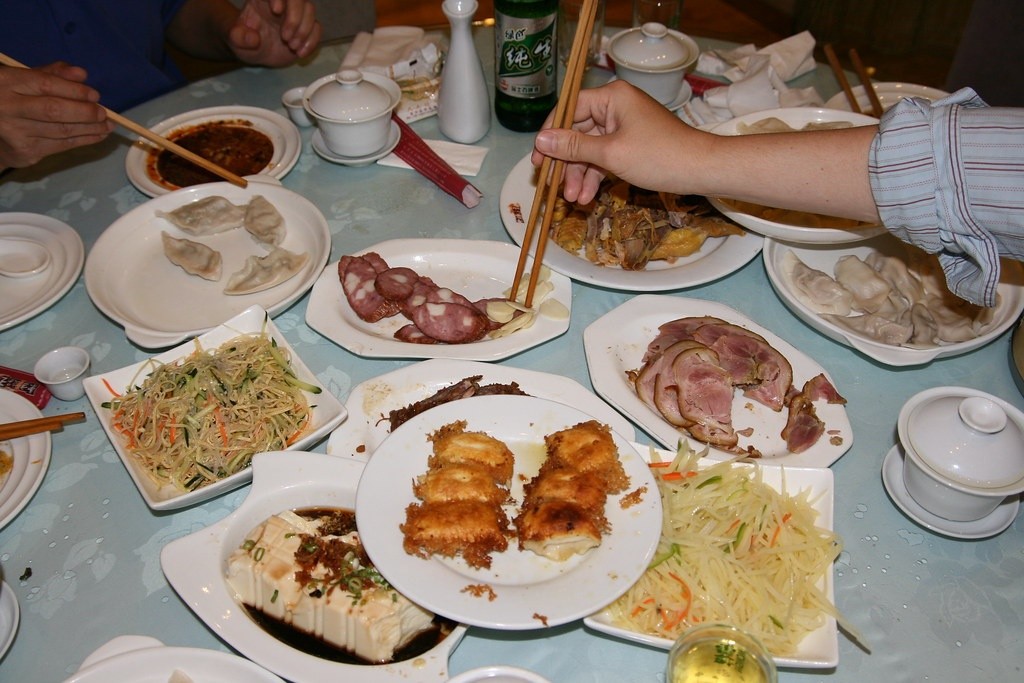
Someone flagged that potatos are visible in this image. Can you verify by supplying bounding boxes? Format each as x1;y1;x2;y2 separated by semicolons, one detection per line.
598;436;869;665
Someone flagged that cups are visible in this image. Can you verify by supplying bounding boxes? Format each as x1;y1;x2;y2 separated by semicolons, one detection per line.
666;624;778;683
35;347;90;401
897;386;1024;521
303;71;402;158
282;87;315;127
607;22;699;106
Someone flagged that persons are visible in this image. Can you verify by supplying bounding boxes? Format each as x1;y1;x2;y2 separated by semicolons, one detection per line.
0;0;322;169
531;78;1024;309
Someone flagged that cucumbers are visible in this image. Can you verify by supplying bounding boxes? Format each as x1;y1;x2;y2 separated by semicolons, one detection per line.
99;310;322;492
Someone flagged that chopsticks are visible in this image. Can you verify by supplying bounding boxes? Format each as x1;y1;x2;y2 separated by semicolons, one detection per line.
824;44;884;120
0;413;86;440
0;53;249;189
510;0;598;309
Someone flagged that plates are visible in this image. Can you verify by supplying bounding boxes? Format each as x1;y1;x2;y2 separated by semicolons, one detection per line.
500;151;763;292
126;106;302;198
607;75;692;111
305;238;571;362
763;236;1024;367
882;442;1020;539
706;107;889;243
0;212;84;332
312;120;401;167
0;388;51;529
824;83;951;119
0;294;855;683
84;181;331;349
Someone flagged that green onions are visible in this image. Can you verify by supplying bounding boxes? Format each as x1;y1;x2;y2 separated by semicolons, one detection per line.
241;534;398;606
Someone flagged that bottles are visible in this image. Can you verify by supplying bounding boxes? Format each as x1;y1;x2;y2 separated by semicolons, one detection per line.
495;0;558;133
439;0;491;144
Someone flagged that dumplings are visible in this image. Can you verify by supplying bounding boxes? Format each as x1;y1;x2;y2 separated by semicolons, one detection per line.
222;249;310;295
156;197;247;235
732;117;876;229
160;231;223;283
780;244;1004;350
243;195;286;251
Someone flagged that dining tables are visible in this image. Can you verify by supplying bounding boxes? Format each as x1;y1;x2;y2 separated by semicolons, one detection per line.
0;28;1024;683
